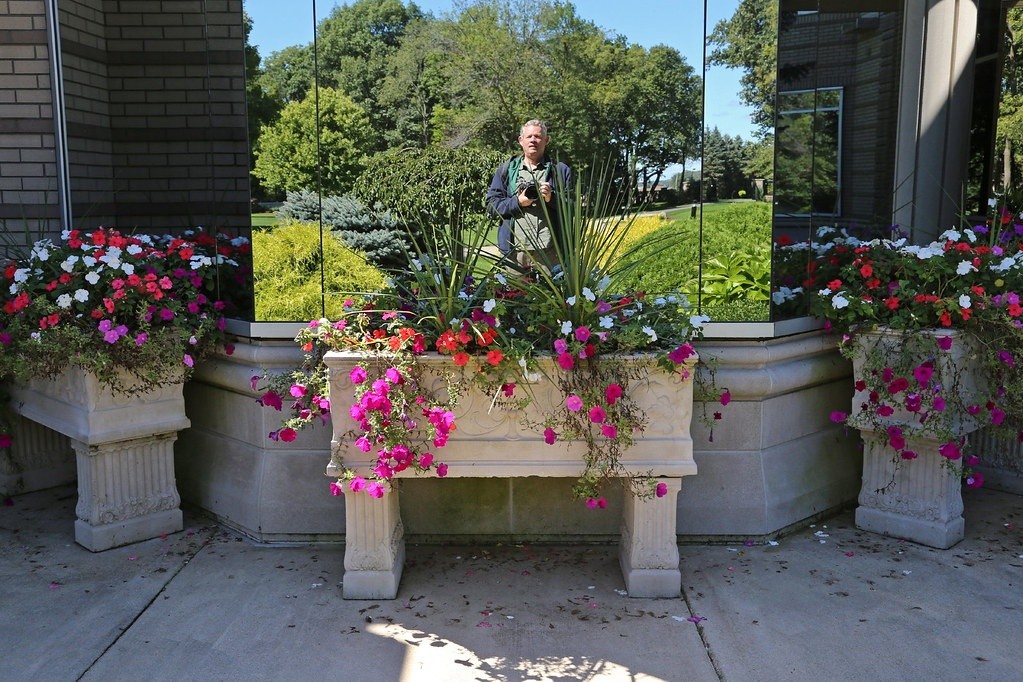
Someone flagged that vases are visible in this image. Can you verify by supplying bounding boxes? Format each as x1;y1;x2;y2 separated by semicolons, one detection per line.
323;349;700;599
843;324;1012;550
10;350;190;552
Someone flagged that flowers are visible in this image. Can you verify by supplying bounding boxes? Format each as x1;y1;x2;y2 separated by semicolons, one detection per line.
245;162;731;511
770;206;1023;488
0;220;242;409
182;224;251;303
773;220;861;317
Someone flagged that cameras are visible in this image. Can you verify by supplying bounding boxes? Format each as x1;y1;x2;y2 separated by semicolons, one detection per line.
520;180;540;199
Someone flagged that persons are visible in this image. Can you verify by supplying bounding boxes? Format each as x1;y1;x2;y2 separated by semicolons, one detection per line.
487;119;574;271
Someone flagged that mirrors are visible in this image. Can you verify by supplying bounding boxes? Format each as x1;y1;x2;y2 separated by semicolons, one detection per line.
203;0;895;339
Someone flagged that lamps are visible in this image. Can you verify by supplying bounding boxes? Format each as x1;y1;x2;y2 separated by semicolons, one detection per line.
620;206;625;219
691;204;697;218
